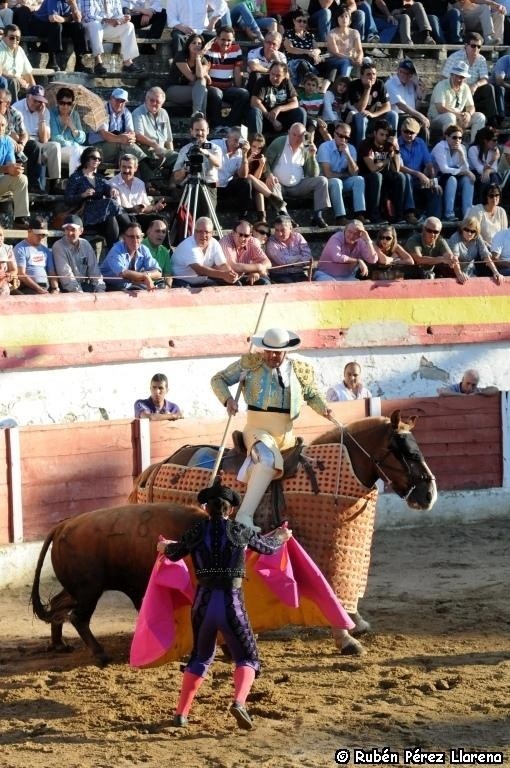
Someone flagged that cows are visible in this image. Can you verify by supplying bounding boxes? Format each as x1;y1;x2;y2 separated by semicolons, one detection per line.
28;503;232;665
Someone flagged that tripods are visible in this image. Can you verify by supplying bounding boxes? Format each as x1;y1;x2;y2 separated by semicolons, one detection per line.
169;172;224;240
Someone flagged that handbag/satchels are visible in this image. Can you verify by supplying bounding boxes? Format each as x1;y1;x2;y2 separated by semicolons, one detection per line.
52;203;85;230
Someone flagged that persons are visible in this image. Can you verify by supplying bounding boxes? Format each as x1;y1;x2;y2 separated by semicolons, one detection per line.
133;373;185;421
211;327;332;534
154;475;292;730
438;368;498;397
326;362;372;402
0;0;510;295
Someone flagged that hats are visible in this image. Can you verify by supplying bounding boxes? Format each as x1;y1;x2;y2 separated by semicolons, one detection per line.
398;60;416;74
197;475;242;506
249;327;302;352
26;85;47;104
62;214;83;229
30;215;50;235
111;88;129;102
449;60;472;78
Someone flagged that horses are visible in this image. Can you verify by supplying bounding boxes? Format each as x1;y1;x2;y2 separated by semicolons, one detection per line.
126;409;437;656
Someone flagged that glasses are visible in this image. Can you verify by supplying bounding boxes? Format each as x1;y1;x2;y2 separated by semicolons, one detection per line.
90;155;101;161
471;44;481;48
8;35;20;41
296;18;307;23
58;100;72;106
237;130;500;240
455;93;459;108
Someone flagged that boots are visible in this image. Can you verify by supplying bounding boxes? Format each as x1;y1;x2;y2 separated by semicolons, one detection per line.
235;463;276;533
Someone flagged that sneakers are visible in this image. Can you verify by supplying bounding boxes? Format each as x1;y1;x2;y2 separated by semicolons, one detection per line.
484;36;500;46
192;110;204;118
365;34;436;57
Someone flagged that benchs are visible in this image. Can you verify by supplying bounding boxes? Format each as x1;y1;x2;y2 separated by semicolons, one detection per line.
0;29;472;239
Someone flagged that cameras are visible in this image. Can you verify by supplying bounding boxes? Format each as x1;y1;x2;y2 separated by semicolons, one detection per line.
238;137;248;148
253;154;264;160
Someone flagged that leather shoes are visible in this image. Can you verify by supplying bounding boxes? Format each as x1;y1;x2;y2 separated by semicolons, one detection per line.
147;186;161;196
46;63;60;71
12;220;31;230
73;64;93;73
93;63;107;75
48;186;66;194
173;715;188;726
229;702;252;729
122;64;145;72
141;156;166;180
